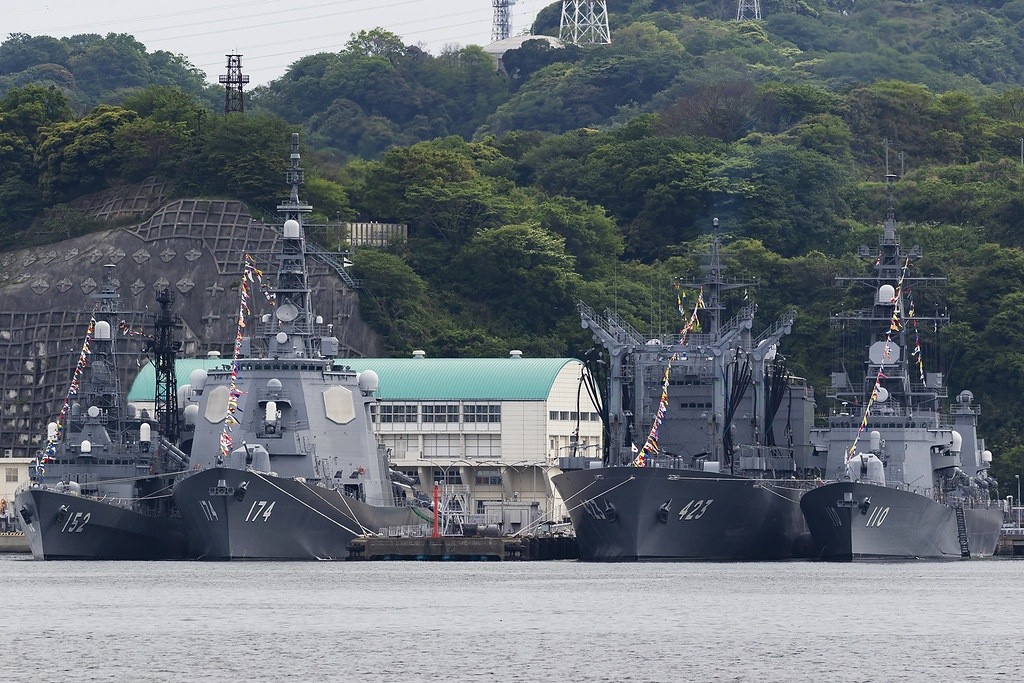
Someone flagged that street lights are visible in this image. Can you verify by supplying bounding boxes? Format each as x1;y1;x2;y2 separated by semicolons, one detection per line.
450;459;504;508
1015;474;1020;529
475;460;528;533
418;457;472;509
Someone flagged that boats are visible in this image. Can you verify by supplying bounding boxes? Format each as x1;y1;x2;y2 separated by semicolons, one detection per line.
14;253;183;562
168;131;429;562
798;139;1003;563
550;218;820;562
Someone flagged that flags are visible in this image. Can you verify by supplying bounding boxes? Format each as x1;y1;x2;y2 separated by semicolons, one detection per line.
907;290;926;386
239;254;276;328
219;331;244;457
37;403;69;480
634;353;676;467
69;317;96;393
675;285;705;347
890;258;908;331
849;335;892;459
